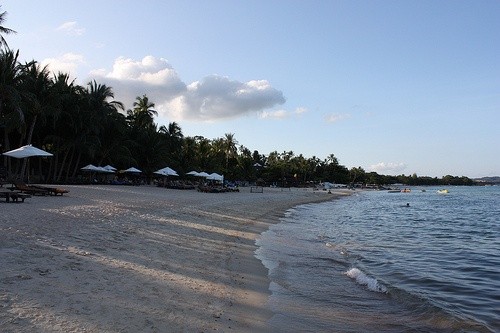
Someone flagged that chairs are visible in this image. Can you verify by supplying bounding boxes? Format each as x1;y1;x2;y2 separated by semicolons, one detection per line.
0;179;71;203
156;181;240;193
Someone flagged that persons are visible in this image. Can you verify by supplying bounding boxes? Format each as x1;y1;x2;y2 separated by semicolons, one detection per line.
406;203;410;207
300;180;331;194
83;174;242;192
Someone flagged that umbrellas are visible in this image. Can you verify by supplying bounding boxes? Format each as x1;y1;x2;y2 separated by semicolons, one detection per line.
82;164;223;182
2;145;54;184
